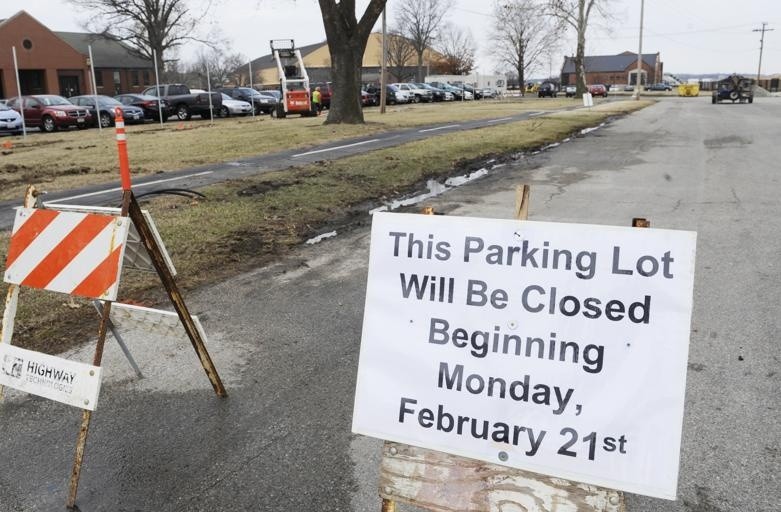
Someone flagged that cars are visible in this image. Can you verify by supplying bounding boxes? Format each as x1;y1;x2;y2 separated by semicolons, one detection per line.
590;85;607;97
643;83;672;91
623;85;634;90
565;87;576;97
360;81;497;105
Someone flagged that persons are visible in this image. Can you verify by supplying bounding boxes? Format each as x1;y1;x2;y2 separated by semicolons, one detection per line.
312;87;322;115
